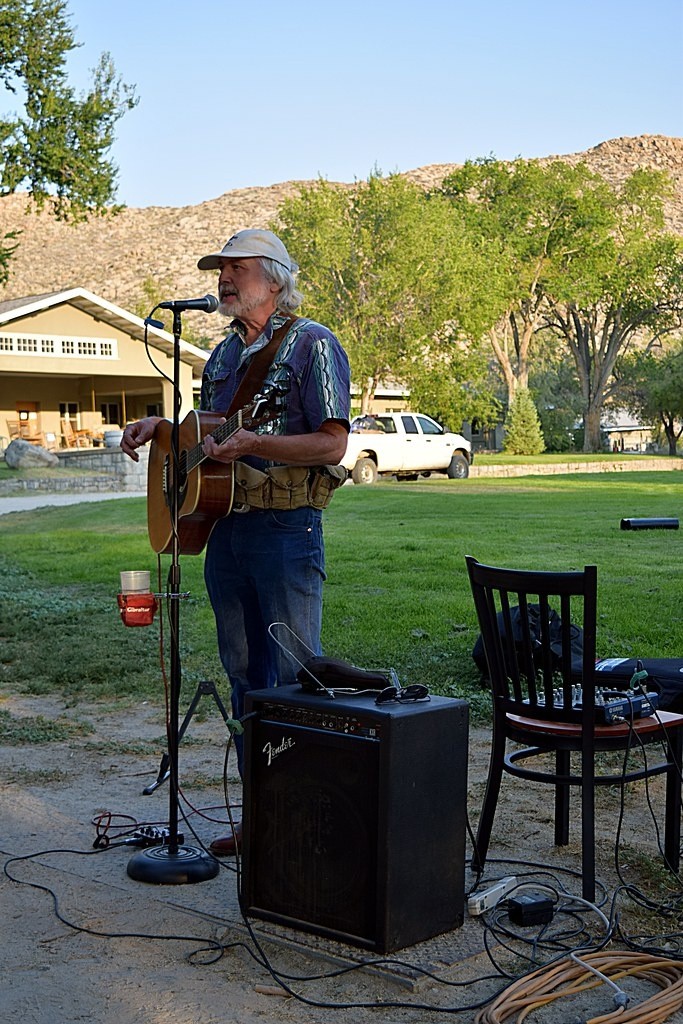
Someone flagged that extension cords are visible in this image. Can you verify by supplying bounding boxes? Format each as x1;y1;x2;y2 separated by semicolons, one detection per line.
469;876;519;915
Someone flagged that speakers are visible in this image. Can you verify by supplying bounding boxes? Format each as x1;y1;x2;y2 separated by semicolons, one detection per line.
245;684;470;953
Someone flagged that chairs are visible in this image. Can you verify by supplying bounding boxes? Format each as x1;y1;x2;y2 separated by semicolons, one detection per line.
61;418;92;448
463;555;683;903
7;419;44;448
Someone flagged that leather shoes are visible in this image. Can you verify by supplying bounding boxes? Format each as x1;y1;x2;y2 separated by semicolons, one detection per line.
209;823;243;856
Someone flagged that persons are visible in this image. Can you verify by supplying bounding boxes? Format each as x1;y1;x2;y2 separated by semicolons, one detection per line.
119;229;351;856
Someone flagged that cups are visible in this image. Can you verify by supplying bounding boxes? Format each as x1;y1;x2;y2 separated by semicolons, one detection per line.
120;571;150;594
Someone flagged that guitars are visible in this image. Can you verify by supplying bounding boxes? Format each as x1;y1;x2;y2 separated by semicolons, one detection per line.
145;382;292;557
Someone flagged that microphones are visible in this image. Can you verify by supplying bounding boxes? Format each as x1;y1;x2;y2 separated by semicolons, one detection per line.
159;295;219;313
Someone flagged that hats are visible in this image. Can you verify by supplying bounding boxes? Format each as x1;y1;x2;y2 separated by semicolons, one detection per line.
197;229;291;271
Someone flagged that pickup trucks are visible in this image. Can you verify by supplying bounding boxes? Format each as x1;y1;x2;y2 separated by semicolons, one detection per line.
335;412;473;484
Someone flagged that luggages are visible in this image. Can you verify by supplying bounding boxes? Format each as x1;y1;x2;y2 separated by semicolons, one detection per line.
594;658;683;714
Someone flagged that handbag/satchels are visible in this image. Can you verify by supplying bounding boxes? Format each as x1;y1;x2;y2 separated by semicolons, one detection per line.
232;462;345;508
471;602;586;679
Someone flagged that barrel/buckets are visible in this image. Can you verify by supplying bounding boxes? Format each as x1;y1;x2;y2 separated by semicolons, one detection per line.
105;431;123;447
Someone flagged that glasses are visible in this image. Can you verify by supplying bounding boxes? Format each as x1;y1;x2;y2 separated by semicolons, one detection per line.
375;684;432;705
